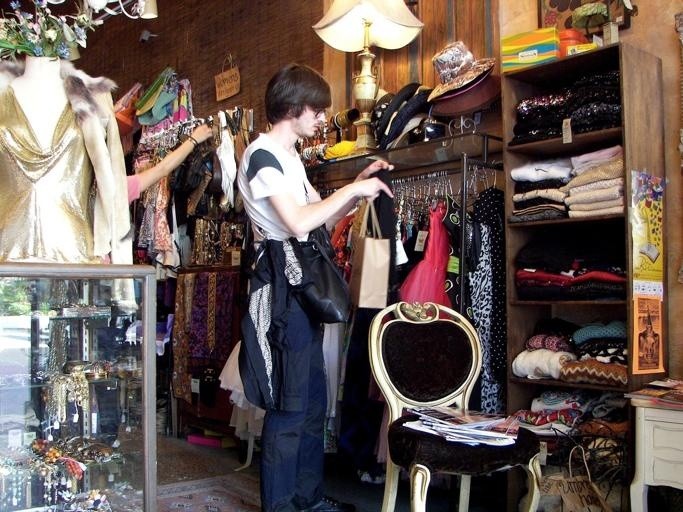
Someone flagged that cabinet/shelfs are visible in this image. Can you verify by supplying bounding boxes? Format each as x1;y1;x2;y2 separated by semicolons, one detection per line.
0;261;158;512
500;41;667;512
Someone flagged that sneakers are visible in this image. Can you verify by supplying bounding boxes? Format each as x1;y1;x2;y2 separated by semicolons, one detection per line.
301;493;356;512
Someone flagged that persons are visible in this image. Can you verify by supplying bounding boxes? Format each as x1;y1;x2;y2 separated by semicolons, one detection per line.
237;64;394;511
0;55;133;266
126;123;213;207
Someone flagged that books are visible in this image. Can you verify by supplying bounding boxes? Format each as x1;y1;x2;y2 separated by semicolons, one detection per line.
624;378;683;405
402;408;520;447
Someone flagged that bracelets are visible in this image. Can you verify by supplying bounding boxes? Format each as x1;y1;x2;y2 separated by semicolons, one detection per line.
187;136;199;145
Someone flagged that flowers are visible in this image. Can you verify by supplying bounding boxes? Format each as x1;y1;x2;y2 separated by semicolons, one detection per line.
1;0;145;62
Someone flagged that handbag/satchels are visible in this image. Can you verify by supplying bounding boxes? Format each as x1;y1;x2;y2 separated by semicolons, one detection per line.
295;243;353;323
532;445;616;512
303;182;336;261
348;200;391;310
114;82;145;134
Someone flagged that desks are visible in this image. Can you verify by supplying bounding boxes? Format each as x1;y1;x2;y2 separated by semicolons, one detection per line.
629;380;683;512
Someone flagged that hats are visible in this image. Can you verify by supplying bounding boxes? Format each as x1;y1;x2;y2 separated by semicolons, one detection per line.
427;40;496;103
369;82;435;152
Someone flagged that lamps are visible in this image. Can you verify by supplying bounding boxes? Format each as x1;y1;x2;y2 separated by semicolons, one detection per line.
311;0;425;157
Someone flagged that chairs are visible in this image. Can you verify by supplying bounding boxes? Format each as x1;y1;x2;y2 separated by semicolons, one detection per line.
366;301;543;512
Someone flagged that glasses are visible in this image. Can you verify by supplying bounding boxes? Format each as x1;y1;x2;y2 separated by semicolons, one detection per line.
315;109;328;118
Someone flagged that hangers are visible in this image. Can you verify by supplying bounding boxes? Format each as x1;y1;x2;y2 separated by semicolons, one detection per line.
317;160;504;220
